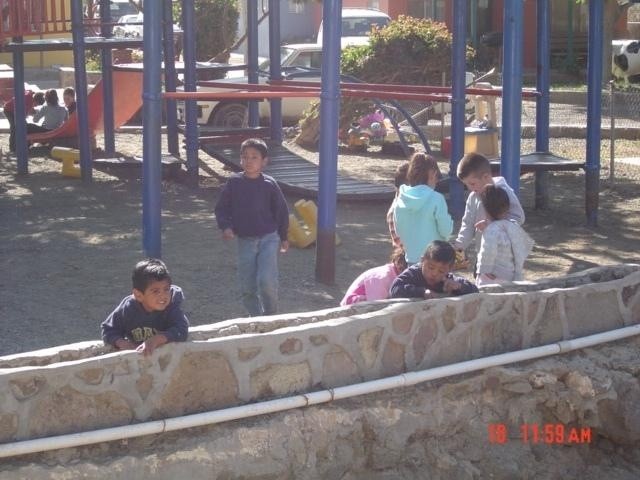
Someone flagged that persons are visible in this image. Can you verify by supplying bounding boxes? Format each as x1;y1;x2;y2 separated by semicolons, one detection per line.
213;136;290;319
385;163;409;254
453;152;527;278
392;152;456;269
476;185;535;284
99;255;192;355
63;88;77;117
388;240;478;302
2;89;45;156
25;90;69;138
336;246;410;305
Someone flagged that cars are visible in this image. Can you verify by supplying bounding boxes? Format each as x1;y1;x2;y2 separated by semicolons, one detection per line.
113;15;180;37
175;6;393;127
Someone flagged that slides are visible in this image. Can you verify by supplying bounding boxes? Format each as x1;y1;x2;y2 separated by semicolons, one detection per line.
24;71;144;145
